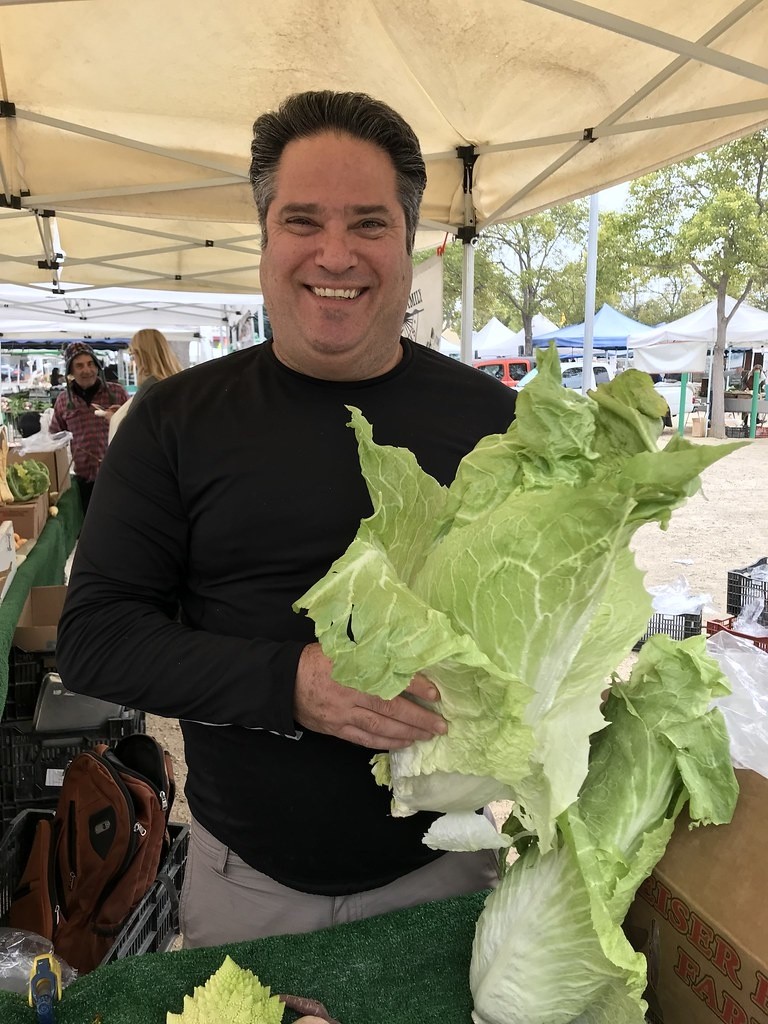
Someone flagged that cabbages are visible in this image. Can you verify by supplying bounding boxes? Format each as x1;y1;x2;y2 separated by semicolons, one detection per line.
292;341;752;1024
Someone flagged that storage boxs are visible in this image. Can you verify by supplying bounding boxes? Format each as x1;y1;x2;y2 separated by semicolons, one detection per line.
621;767;768;1023
0;445;72;604
726;426;768;438
0;809;191;969
707;616;768;653
727;557;768;626
0;582;146;825
632;595;702;652
692;418;705;437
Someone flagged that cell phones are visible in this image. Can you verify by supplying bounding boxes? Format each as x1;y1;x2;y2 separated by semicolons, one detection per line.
91;403;106;412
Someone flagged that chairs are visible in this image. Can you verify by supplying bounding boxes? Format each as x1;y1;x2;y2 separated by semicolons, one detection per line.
685;403;711;428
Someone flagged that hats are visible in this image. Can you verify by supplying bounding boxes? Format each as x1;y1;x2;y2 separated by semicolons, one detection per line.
64;341;116;409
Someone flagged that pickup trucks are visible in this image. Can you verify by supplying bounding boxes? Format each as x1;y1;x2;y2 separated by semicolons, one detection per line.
472;353;696;436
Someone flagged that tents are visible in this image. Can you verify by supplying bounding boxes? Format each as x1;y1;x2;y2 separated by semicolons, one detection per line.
0;212;768;435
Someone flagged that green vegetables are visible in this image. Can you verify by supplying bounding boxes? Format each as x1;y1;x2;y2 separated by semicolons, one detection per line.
8;399;52;419
5;459;51;502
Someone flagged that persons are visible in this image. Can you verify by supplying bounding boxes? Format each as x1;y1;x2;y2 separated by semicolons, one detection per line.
48;342;130;518
96;329;182;422
57;92;518;952
740;363;767;427
50;368;66;409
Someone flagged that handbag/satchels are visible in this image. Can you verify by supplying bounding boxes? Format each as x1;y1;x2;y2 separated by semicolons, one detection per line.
8;734;176;975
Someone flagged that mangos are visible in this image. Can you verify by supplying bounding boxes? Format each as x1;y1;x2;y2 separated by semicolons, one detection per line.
49;507;59;516
14;533;28;550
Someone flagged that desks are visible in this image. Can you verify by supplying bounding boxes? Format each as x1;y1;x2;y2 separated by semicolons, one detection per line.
725;398;768;427
0;475;84;715
